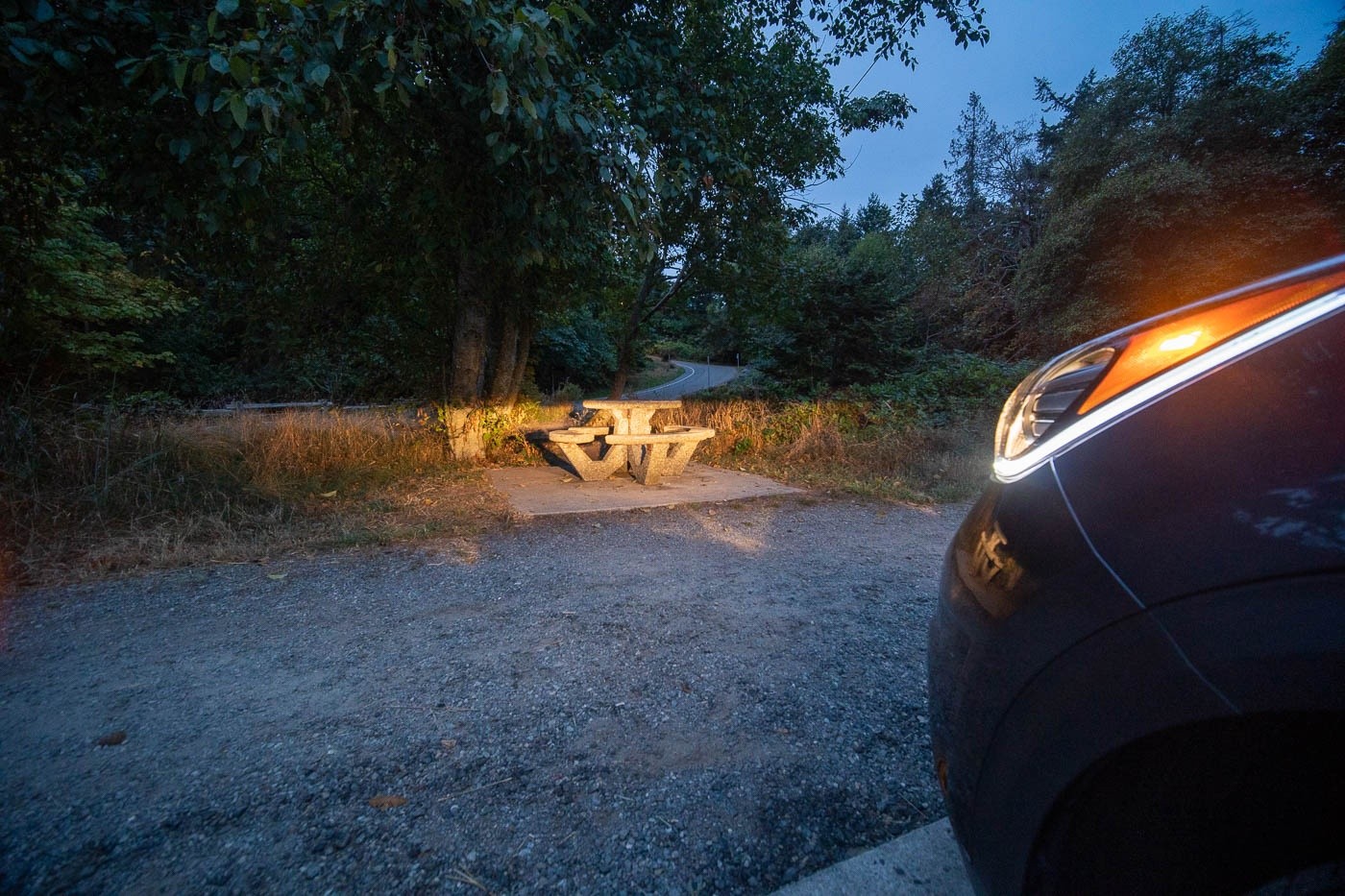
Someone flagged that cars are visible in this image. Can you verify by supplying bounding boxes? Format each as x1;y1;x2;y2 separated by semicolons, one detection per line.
924;253;1345;896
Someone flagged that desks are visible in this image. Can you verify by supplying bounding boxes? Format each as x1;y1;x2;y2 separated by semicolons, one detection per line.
582;399;683;476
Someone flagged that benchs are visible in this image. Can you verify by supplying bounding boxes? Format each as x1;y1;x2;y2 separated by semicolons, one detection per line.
549;424;716;484
